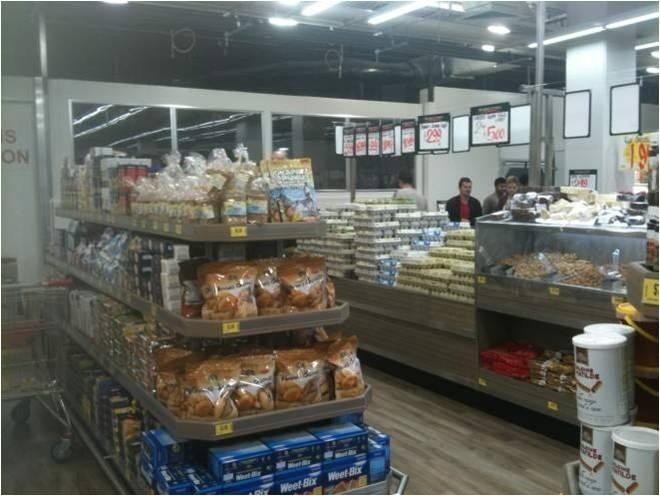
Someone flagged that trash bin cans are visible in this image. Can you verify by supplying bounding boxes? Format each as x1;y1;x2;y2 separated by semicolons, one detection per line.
0;257;18;317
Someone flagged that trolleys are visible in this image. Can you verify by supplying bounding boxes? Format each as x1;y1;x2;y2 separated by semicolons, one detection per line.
2;279;77;462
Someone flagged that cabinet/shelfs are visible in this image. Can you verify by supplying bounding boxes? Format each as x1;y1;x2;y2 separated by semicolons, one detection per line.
44;207;410;495
475;210;647;427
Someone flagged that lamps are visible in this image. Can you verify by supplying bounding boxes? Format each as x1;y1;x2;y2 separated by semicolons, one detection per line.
367;1;659;74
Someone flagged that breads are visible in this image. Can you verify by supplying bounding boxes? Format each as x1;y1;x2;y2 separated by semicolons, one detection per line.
157;286;363;418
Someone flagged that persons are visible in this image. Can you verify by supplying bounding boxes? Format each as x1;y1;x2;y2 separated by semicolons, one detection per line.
393;170;429;210
446;177;482;227
482;176;508;215
498;175;520;210
